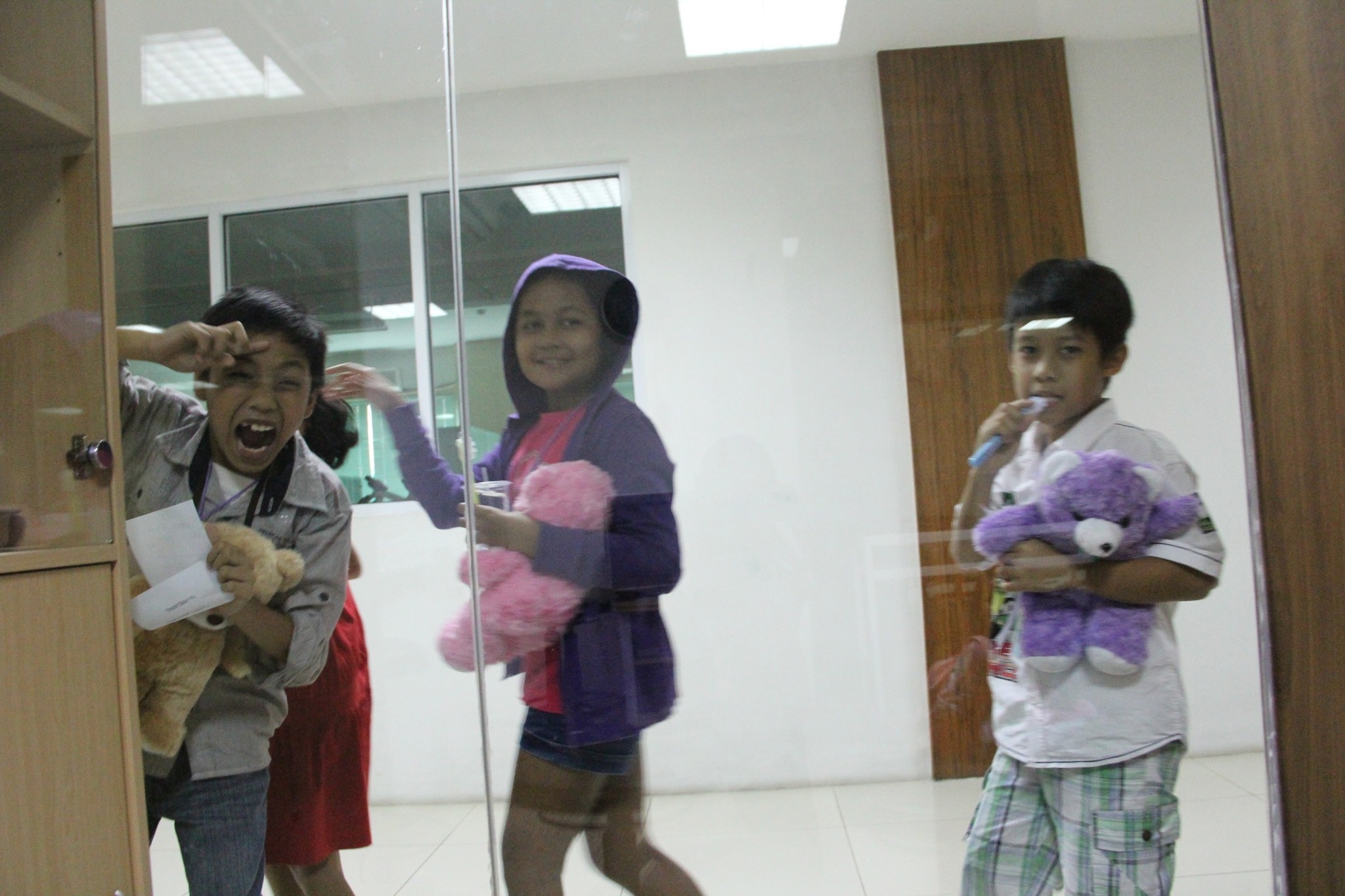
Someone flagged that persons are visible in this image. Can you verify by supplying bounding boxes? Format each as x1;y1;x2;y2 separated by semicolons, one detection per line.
263;378;373;896
112;283;354;896
946;257;1226;896
315;254;703;896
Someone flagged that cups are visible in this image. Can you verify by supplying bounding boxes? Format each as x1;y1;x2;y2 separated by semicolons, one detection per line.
461;481;511;552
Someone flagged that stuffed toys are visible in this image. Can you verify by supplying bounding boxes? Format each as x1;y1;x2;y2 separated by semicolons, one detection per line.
127;521;306;758
435;458;619;674
969;451;1200;678
